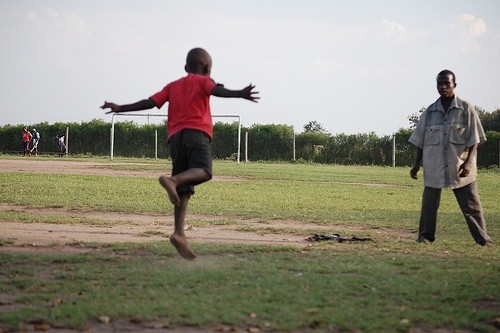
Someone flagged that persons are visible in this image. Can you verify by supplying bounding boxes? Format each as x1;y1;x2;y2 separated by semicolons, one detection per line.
100;47;260;260
22;126;39;156
56;131;65;157
408;70;495;247
